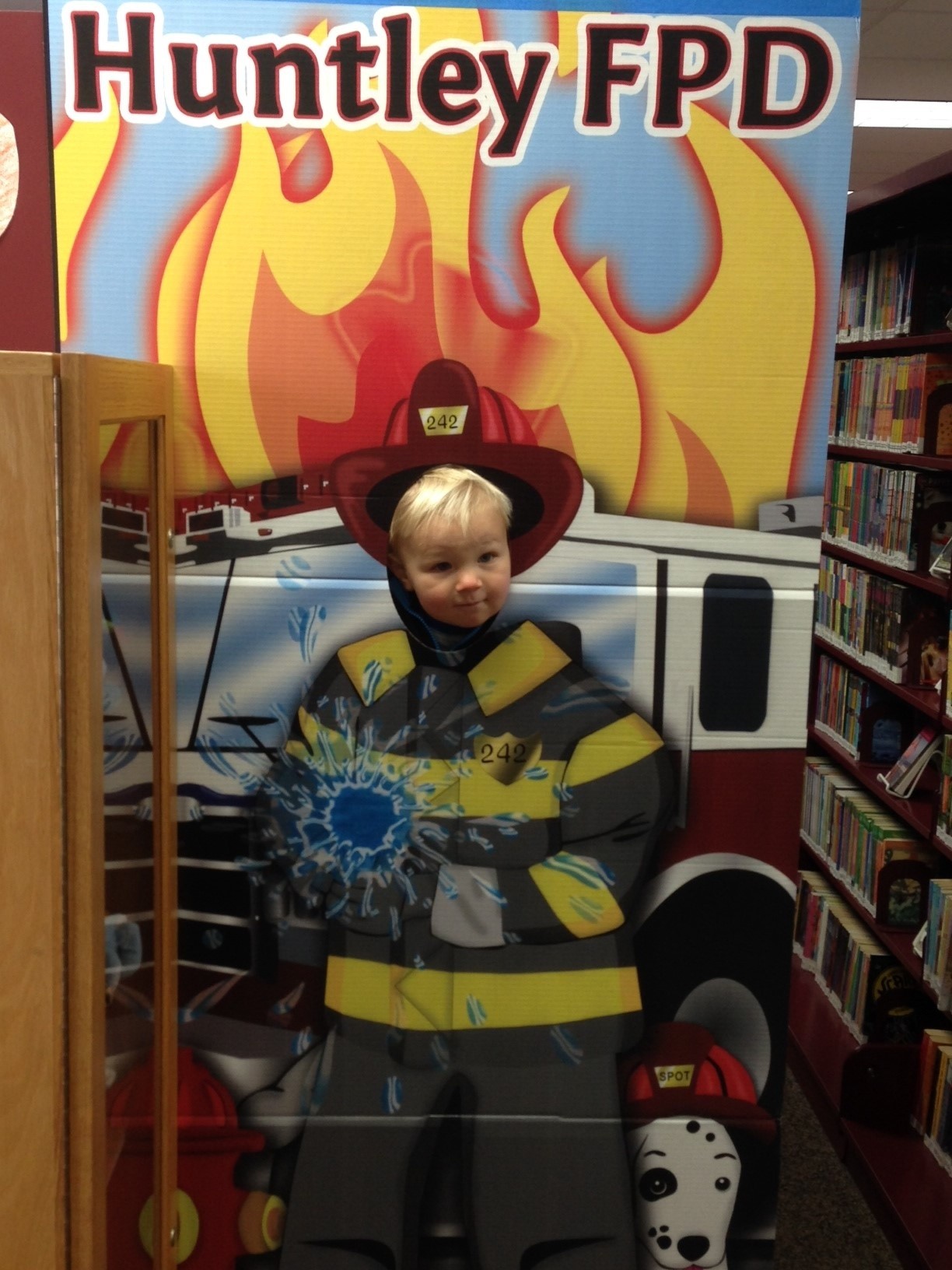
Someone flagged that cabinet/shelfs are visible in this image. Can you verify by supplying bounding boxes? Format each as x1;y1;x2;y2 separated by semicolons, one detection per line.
783;147;952;1270
0;346;182;1270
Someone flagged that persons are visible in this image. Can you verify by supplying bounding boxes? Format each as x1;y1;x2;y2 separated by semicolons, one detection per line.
391;460;513;633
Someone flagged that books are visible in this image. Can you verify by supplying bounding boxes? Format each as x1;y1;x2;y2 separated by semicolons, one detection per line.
774;236;952;1179
